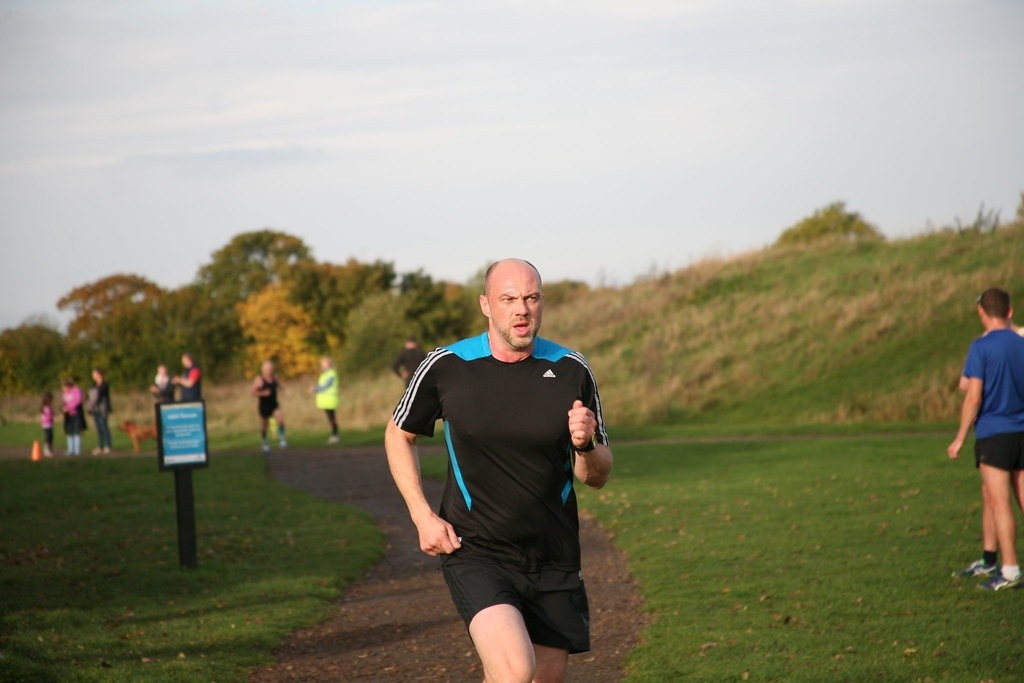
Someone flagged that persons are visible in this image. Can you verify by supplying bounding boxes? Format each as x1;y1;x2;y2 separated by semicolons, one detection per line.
86;367;113;456
40;392;56;458
60;381;88;455
150;364;174;402
392;337;427;387
947;289;1024;591
308;356;339;445
251;361;288;454
172;353;201;402
384;259;613;683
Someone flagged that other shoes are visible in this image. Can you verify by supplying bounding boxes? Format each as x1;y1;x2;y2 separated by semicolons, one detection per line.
278;438;287;449
261;443;270;455
327;434;338;446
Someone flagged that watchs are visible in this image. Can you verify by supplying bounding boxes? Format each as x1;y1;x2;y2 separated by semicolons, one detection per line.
573;433;597;452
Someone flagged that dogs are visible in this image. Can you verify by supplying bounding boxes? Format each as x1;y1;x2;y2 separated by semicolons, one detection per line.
118;419;157;453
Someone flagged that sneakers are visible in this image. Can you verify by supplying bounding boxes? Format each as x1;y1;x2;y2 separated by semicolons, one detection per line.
951;559;999;583
976;570;1024;593
